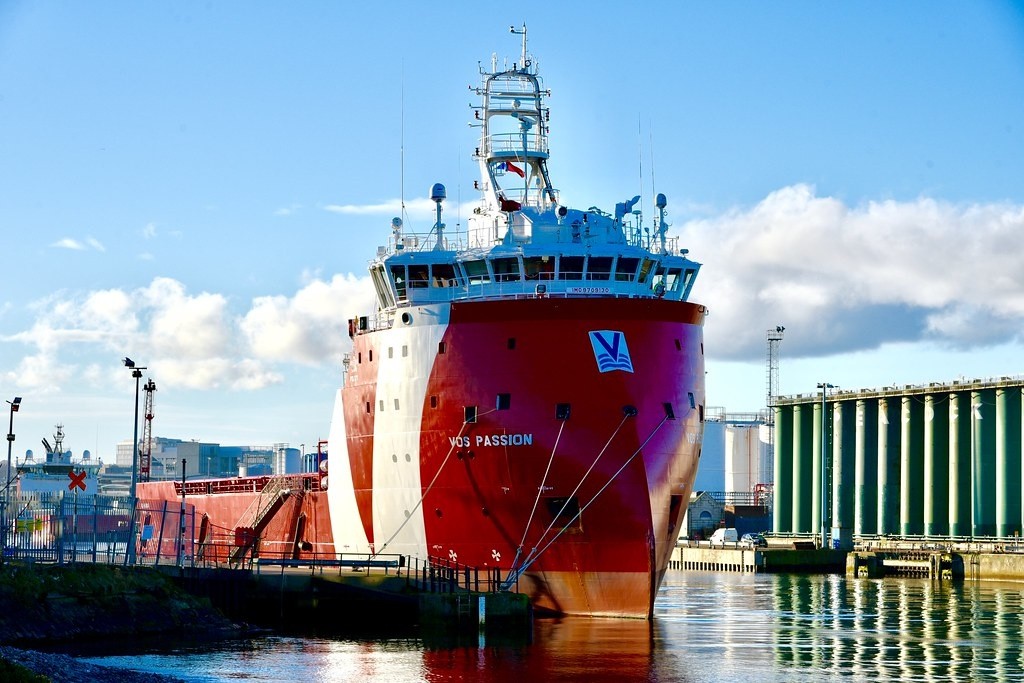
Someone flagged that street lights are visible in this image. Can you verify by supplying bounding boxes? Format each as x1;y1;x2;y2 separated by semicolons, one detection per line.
816;382;835;547
5;397;23;536
121;357;148;564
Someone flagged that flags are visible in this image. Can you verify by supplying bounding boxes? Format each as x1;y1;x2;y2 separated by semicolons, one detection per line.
491;162;507;169
508;162;524;178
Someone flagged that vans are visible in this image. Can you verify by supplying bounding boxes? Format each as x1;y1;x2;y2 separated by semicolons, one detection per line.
710;527;738;543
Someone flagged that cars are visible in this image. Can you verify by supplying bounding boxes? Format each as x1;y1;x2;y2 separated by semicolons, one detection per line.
742;533;769;548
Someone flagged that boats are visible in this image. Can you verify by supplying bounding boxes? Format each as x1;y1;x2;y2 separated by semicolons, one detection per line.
131;20;707;618
